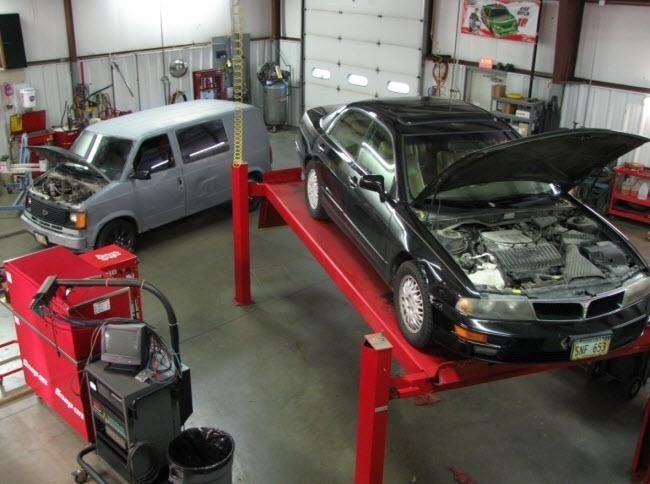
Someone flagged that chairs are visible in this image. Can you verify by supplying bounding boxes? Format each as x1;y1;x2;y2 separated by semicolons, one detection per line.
334;118;521;198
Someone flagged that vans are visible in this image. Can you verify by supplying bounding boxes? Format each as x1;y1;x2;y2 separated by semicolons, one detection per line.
11;97;274;253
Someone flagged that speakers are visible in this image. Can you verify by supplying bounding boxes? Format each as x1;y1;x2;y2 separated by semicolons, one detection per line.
0;14;27;69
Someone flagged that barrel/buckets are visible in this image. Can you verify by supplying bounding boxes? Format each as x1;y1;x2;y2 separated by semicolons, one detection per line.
192;69;227;100
53;123;79;150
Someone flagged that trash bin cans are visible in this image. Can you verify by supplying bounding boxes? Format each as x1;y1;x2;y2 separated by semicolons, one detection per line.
166;428;234;484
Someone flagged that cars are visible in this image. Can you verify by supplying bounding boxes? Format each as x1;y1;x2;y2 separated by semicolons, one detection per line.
480;3;518;38
291;93;650;368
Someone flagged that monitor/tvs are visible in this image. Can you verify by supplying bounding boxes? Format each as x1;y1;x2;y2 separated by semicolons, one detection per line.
100;322;151;376
